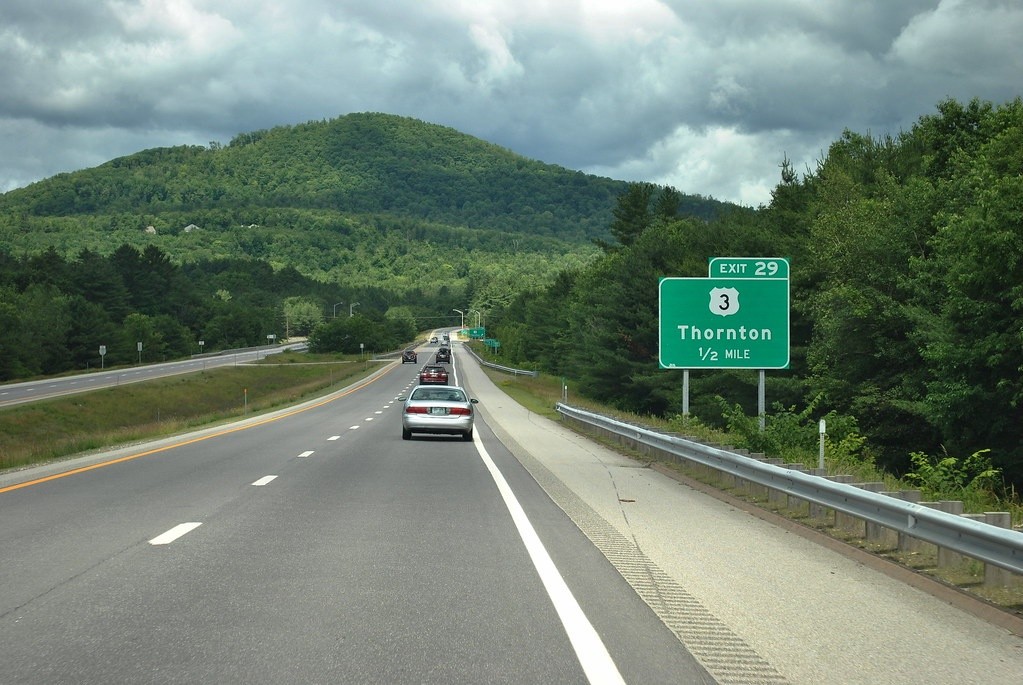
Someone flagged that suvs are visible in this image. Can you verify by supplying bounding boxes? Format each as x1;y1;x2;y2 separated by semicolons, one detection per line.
418;364;450;385
402;350;418;365
435;351;450;363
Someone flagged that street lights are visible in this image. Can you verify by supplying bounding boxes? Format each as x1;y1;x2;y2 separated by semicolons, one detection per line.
453;309;464;336
349;302;360;317
465;308;481;340
333;302;342;319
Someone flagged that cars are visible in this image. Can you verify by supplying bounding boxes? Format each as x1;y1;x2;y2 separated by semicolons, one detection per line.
431;336;438;343
397;383;479;442
440;340;448;346
